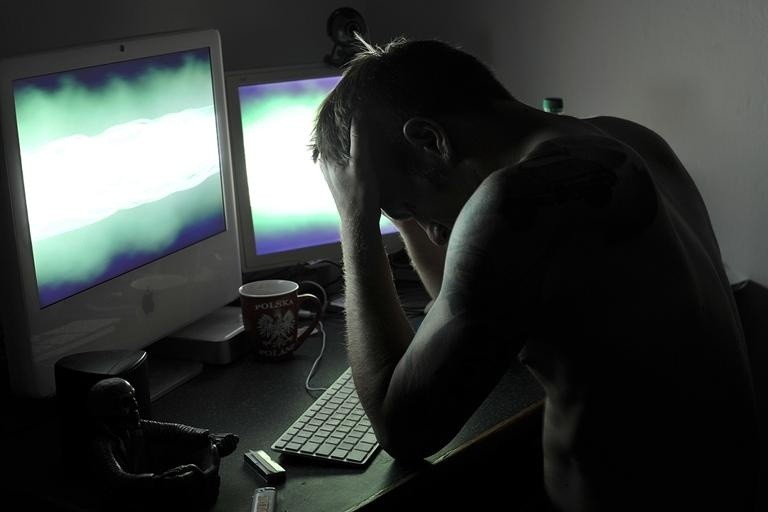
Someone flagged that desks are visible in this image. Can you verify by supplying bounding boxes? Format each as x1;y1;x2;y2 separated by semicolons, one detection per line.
0;273;546;511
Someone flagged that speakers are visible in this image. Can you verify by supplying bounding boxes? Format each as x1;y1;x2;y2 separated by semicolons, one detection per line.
54;351;152;512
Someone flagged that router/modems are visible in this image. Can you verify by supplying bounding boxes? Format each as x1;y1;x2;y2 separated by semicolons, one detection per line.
168;306;252;366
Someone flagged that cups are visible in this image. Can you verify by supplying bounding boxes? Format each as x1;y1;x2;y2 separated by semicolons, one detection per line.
236;279;329;358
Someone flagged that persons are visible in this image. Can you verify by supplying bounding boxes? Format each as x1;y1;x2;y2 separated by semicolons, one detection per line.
307;32;755;510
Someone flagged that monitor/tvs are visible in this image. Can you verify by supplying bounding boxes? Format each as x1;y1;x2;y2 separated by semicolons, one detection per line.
224;61;406;310
0;27;243;406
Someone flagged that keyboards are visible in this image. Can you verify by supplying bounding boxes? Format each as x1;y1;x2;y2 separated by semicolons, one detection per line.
270;363;380;468
